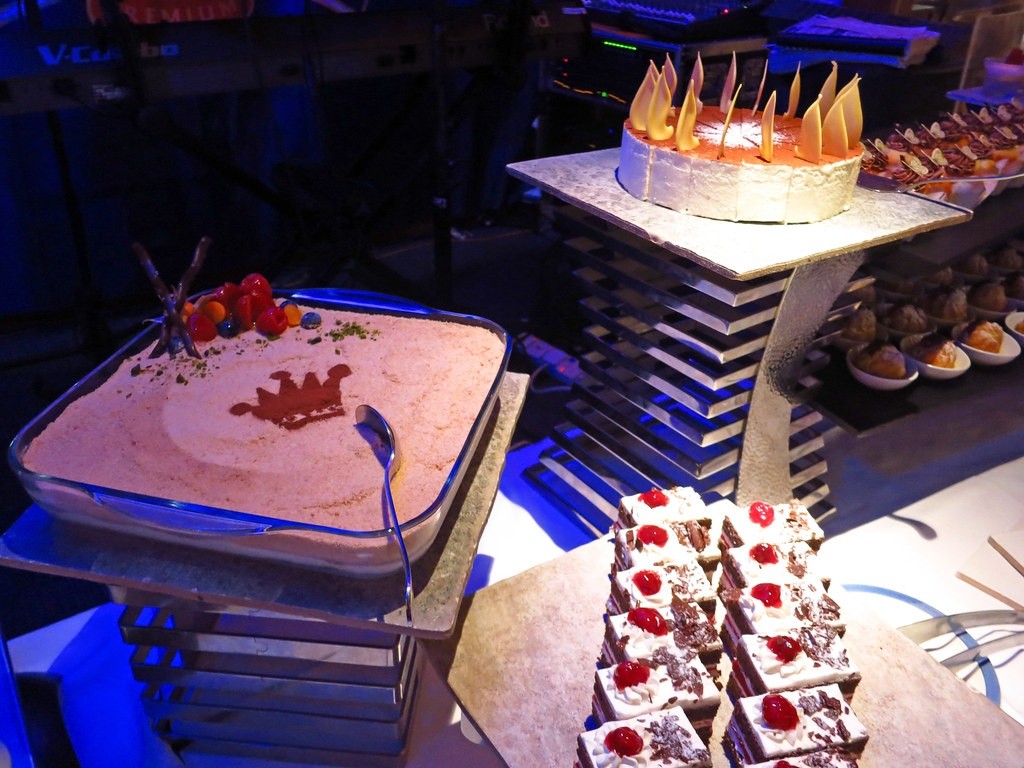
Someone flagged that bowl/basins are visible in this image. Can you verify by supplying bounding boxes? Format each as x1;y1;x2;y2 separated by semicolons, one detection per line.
840;328;889;348
920;309;977;330
1003;297;1024;310
875;317;938;340
9;286;515;579
901;334;971;380
967;303;1018;319
952;322;1021;366
1004;312;1024;346
846;343;919;390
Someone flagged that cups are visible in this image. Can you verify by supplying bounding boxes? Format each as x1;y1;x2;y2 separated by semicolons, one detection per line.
983;56;1024;97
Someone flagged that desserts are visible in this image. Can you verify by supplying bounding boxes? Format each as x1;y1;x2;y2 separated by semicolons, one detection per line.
861;96;1024;211
839;238;1024;380
574;485;870;768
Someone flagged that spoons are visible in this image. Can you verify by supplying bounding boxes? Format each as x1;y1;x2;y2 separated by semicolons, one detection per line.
355;404;414;628
856;171;1024;194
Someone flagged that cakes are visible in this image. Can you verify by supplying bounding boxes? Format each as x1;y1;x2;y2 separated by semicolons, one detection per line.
617;50;865;225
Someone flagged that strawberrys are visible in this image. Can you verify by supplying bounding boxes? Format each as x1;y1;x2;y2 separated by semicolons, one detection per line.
185;272;288;340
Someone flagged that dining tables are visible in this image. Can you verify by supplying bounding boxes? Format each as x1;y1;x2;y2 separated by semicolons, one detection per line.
0;383;1024;768
